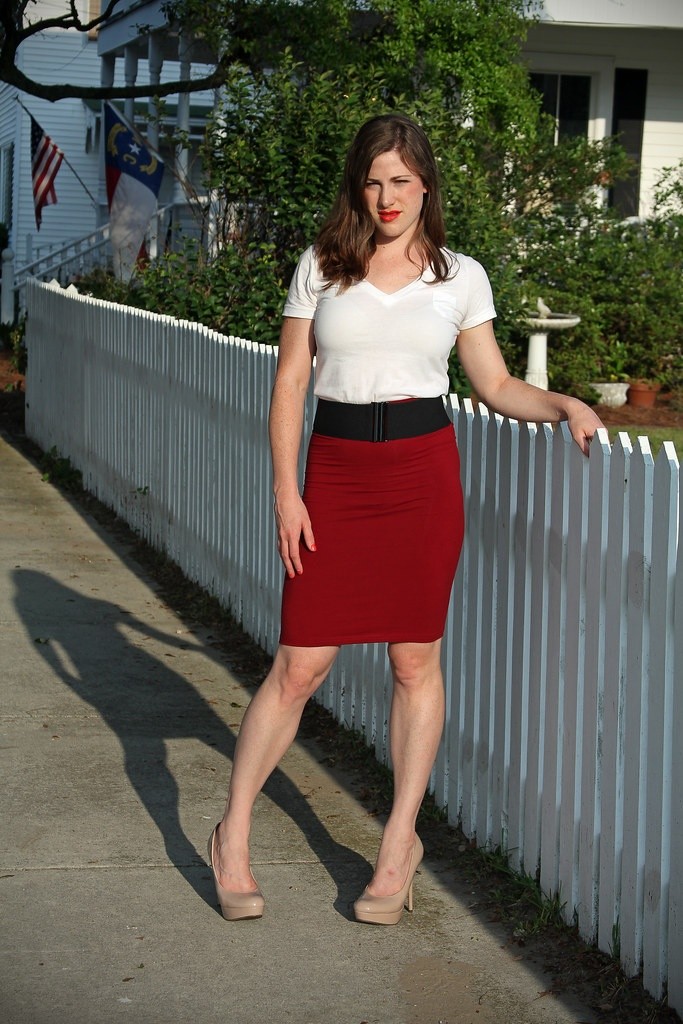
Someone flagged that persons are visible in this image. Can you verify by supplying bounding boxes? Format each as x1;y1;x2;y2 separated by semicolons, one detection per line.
206;113;609;928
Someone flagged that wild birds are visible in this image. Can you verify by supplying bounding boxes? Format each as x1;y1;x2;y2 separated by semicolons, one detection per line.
536;297;553;318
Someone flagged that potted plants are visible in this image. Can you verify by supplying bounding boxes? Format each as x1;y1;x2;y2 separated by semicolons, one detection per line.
586;342;668;411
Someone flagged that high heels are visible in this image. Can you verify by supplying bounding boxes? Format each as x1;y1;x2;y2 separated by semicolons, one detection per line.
353;832;424;925
207;822;265;922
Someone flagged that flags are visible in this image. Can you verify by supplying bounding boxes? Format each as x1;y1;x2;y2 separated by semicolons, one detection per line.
103;102;166;298
30;115;65;233
